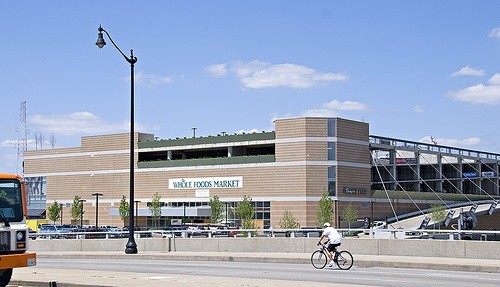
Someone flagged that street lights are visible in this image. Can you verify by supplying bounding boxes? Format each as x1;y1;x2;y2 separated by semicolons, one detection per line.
95;23;107;49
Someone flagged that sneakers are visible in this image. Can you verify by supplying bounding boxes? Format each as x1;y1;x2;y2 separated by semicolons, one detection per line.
329;260;334;267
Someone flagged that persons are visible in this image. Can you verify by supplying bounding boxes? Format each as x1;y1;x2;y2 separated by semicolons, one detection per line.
317;222;342;268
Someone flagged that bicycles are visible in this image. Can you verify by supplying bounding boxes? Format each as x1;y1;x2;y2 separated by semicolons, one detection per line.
311;242;353;270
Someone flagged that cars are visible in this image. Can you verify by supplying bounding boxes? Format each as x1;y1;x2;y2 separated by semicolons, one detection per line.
28;224;148;241
294;226;322;237
149;229;177;238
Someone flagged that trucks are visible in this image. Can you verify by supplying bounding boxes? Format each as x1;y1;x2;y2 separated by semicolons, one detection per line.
26;220;48;234
0;174;36;287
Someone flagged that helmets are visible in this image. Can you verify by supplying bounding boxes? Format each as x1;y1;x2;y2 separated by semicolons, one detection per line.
324;222;330;226
